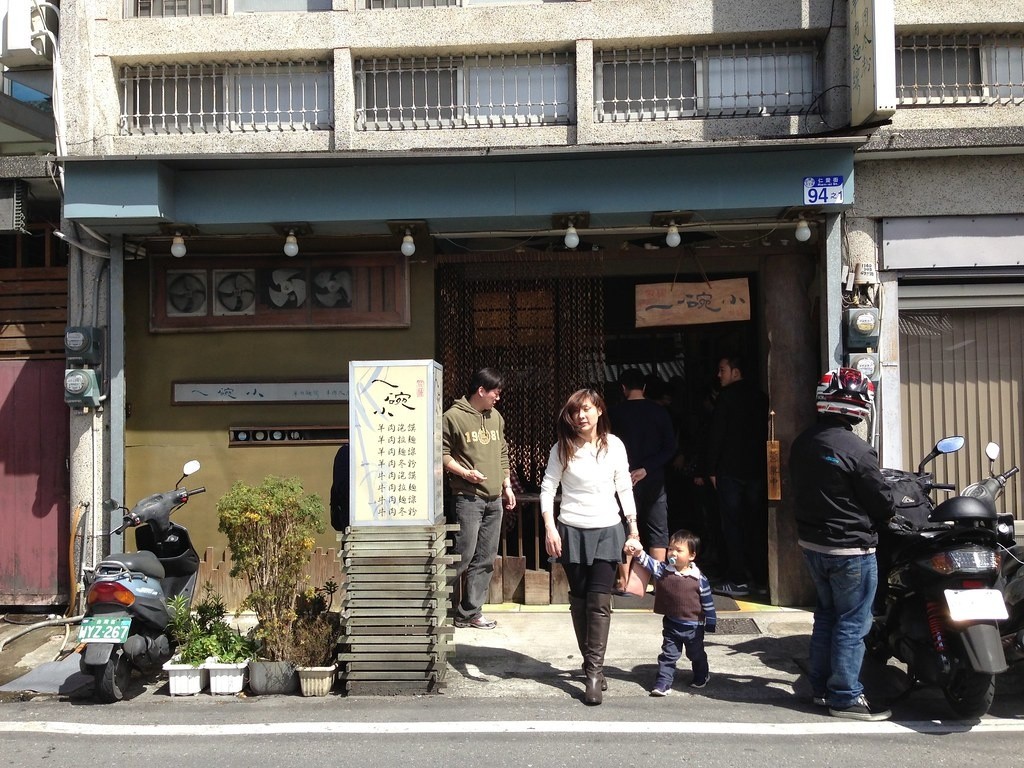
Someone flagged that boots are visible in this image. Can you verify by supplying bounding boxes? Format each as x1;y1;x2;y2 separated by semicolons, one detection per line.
584;590;612;704
566;591;608;691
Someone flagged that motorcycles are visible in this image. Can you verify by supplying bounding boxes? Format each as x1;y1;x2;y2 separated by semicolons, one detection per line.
959;440;1024;663
867;436;1010;720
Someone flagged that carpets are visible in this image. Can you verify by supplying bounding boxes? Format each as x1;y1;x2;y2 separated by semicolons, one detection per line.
611;585;740;611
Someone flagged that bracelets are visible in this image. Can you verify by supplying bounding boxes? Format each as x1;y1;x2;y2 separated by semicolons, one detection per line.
505;485;512;487
626;519;636;523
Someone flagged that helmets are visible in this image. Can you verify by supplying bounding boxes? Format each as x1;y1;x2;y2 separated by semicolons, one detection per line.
815;367;875;420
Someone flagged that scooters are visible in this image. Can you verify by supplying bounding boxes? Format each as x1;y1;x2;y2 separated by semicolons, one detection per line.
79;460;205;702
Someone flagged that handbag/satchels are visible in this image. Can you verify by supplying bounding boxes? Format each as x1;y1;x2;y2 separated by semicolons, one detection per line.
887;477;944;535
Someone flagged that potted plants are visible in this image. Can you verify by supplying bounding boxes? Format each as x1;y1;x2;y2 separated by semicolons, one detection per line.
163;475;340;697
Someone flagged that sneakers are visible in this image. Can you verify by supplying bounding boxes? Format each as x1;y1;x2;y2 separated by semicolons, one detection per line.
814;693;893;720
713;581;748;595
690;671;710;689
454;612;498;630
651;682;670;695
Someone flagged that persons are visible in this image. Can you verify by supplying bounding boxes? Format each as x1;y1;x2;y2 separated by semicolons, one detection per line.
625;530;716;696
790;369;896;721
540;389;639;705
330;444;349;565
442;367;517;629
607;351;769;596
